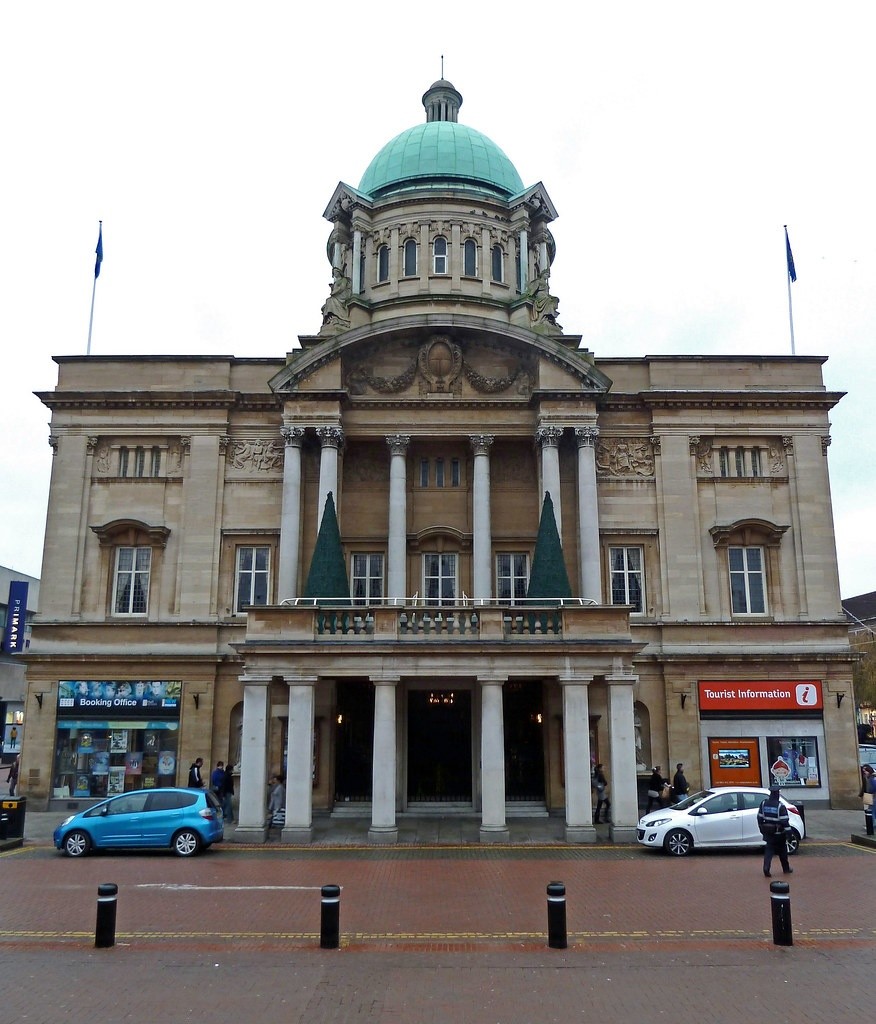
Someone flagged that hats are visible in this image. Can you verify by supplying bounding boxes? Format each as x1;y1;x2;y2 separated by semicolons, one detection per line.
768;786;781;792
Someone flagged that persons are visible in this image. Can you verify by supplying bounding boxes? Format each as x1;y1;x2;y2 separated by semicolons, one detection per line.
188;757;203;788
251;440;264;471
265;440;284;468
269;776;285;826
321;267;350;322
6;754;20;796
235;440;251;466
646;766;671;815
614;438;633;472
527;268;560;325
757;786;793;877
858;765;876;830
10;727;18;748
592;764;610;824
597;439;610;465
212;761;237;824
0;736;4;758
673;764;689;801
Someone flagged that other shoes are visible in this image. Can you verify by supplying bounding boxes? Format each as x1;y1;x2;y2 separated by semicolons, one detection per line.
765;873;771;877
783;868;793;873
605;819;610;823
231;820;237;824
595;821;603;824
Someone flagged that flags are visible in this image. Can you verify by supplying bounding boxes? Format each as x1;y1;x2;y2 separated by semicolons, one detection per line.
787;233;797;283
95;228;103;278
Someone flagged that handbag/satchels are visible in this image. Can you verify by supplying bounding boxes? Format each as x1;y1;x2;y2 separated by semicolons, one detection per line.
591;773;604;792
659;786;670;799
862;793;874;805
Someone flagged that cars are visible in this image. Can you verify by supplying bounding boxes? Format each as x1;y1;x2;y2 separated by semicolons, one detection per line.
858;744;876;780
52;786;225;858
636;786;805;856
